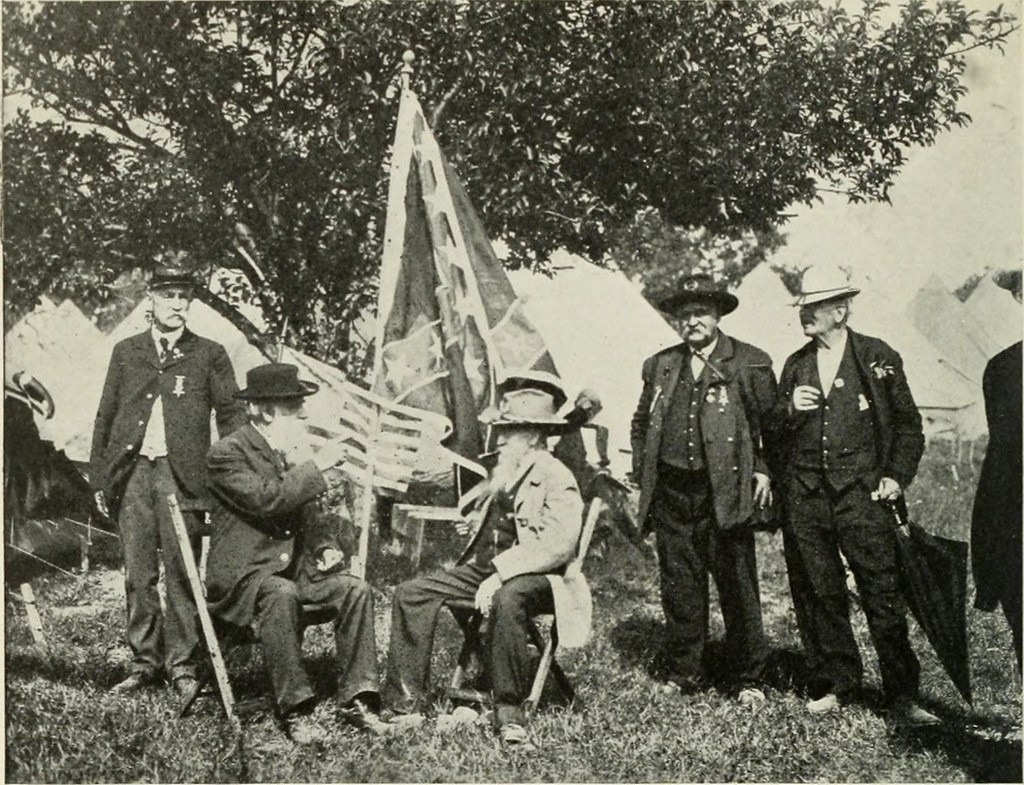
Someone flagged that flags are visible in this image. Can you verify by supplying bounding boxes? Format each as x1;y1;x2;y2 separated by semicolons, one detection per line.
376;88;586;508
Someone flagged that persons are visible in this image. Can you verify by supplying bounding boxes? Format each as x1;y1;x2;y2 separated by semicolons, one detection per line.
389;390;584;743
971;337;1023;687
204;361;391;747
760;261;944;727
88;264;249;698
630;271;778;711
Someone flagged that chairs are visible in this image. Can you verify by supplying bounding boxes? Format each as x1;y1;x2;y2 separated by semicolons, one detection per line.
443;496;601;723
166;493;326;731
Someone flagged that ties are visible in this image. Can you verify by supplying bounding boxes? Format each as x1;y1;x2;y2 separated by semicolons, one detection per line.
160;337;170;359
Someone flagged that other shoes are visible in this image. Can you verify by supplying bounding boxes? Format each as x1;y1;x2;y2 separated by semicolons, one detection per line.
335;700;389;735
379;708;424;726
806;693;852;714
886;699;944;727
174;675;215;695
662;681;683;694
739;687;764;704
109;671;166;695
285;711;326;743
501;722;531;744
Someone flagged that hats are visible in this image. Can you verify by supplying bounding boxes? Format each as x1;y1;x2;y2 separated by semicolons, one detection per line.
231;362;319;398
788;263;861;307
992;254;1024;290
488;388;569;425
149;264;208;290
656;273;738;318
